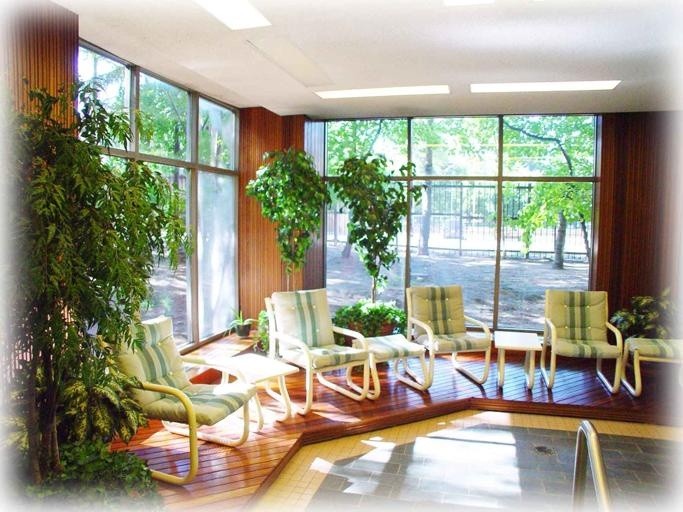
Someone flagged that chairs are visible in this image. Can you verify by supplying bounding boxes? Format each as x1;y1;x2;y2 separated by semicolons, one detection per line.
403;283;493;395
263;284;373;418
537;287;627;396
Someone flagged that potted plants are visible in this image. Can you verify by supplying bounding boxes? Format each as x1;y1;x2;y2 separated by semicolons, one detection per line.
220;307;256;341
321;147;430;370
606;286;679;400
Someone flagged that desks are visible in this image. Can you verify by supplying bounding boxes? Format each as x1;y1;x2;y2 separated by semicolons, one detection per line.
620;338;682;399
208;353;298;432
346;328;430;399
96;310;259;491
495;325;542;393
